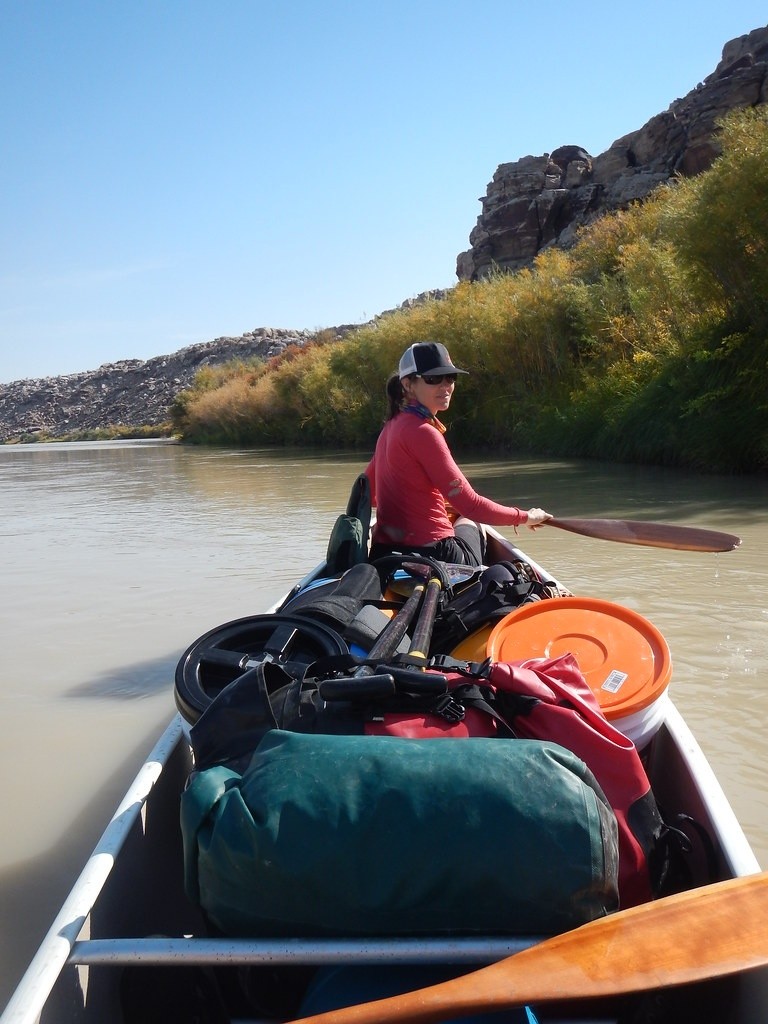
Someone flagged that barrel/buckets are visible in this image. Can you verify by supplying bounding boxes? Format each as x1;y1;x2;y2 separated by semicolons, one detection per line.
487;596;672;786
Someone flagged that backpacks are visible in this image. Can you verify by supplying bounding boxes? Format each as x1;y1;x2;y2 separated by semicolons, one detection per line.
438;559;543;652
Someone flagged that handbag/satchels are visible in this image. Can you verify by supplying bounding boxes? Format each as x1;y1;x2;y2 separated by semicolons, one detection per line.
178;651;713;939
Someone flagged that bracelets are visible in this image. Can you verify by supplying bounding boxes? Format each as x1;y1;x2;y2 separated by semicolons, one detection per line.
513;506;521;535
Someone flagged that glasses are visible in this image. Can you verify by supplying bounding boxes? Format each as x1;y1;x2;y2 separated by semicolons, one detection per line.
416;374;457;385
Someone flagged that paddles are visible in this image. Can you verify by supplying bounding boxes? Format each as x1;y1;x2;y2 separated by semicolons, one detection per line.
541;515;747;557
277;865;768;1024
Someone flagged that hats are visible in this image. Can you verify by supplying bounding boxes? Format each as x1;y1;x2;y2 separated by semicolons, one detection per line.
401;343;470;383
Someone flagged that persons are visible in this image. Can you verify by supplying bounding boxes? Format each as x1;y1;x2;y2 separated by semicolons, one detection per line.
361;341;554;567
